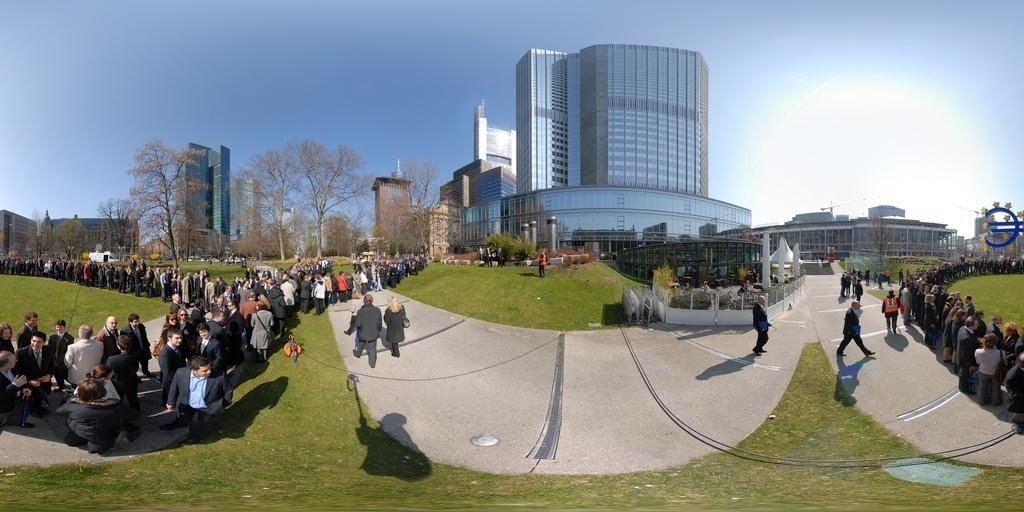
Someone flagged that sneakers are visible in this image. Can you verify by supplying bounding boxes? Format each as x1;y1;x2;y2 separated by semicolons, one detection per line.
391;352;399;357
904;318;924;330
353;349;361;358
344;329;351;335
369;360;375;368
943;359;959;375
886;326;896;333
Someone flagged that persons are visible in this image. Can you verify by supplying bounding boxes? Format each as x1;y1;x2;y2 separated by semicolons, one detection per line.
750;294;773;357
536;249;546;278
833;254;1024;434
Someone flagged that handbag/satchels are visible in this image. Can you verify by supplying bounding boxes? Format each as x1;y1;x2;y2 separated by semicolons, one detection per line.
993;349;1010;384
927;329;939;346
402;317;409;328
266;327;275;344
14;393;30;427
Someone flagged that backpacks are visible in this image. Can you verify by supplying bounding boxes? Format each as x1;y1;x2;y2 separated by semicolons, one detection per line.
968;367;981;394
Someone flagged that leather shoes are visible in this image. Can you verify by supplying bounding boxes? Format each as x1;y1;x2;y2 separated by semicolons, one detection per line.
864;350;876;356
32;406;49;418
836;350;847;356
181;436;200;445
752;347;768;357
16;422;36;428
142;368;152;378
159;416;188;430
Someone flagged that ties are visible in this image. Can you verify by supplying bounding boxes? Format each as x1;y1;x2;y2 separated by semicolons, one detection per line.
55;335;61;358
31;326;37;333
135;330;143;349
34;350;42;371
174;348;178;354
200;339;206;356
